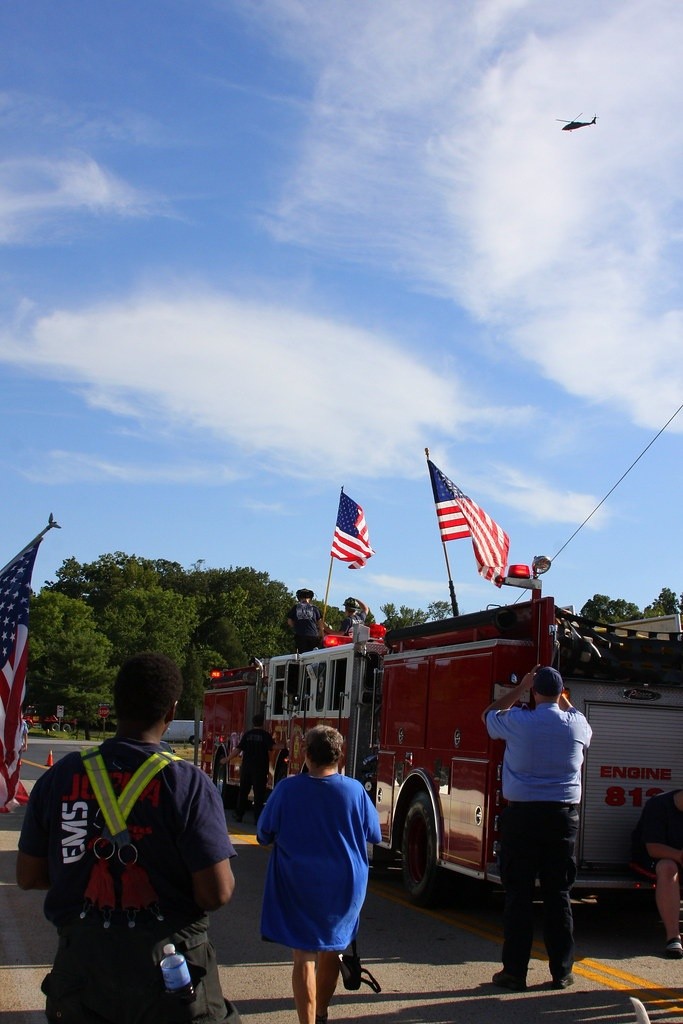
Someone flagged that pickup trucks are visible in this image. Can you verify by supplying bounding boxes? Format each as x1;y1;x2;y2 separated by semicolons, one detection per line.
160;720;203;745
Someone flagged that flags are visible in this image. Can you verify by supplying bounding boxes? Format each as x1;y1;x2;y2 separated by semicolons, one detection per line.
330;492;376;569
427;460;510;588
0;533;44;813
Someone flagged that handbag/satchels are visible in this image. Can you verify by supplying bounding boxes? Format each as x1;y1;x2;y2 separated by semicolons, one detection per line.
340;940;382;993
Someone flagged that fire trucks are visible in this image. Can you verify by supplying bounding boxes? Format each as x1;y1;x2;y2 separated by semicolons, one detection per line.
201;624;388;808
23;704;77;732
372;555;683;910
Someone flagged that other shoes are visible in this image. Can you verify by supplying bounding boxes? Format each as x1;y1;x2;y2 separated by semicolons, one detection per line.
553;974;574;989
492;970;527;991
315;1015;328;1024
664;937;683;958
231;811;243;822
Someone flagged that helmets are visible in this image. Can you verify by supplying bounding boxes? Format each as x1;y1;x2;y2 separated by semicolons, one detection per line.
343;598;361;610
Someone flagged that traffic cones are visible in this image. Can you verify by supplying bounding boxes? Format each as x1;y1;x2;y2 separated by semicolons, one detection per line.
43;749;56;767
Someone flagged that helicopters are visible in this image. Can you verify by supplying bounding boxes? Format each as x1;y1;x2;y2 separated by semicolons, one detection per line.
555;112;599;133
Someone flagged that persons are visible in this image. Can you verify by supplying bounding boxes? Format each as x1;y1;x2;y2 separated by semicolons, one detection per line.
287;588;324;710
220;715;290;826
16;653;242;1024
318;597;368;709
482;668;593;990
256;724;382;1024
21;715;28;752
619;789;683;957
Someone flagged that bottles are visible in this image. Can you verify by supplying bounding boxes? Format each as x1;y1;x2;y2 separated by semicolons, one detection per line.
160;943;194;1001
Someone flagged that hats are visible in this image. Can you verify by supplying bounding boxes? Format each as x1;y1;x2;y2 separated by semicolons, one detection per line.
296;589;314;600
534;667;563;697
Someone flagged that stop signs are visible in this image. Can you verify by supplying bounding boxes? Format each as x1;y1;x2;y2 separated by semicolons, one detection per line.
98;707;110;718
